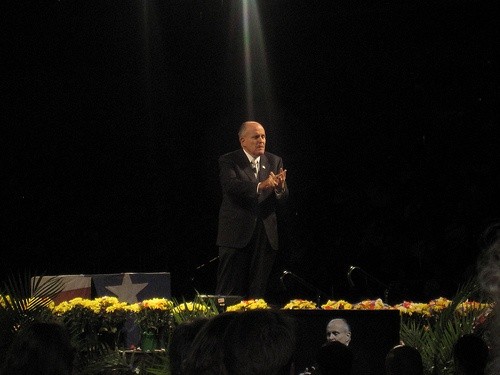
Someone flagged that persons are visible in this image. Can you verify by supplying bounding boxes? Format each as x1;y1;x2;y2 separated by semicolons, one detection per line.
325;316;352;348
166;311;492;375
216;120;290;296
0;322;74;375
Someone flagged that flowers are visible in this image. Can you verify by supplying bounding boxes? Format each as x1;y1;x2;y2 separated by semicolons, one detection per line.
0;293;494;328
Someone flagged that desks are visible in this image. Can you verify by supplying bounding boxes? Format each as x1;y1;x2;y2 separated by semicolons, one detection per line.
168;308;401;375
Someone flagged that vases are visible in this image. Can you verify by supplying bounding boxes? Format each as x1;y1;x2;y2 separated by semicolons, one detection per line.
142;331;159;350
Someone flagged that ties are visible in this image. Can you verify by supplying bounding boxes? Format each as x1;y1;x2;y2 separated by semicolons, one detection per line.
251;159;258;179
